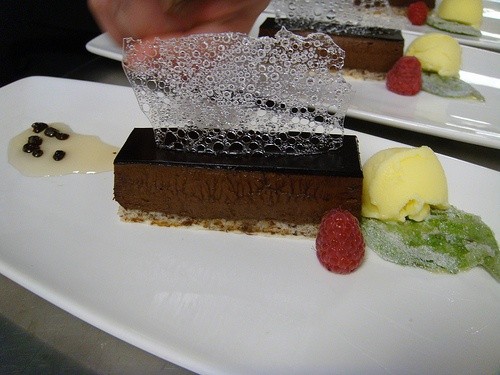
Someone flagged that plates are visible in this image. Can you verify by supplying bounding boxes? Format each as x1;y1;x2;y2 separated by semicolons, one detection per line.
0;76;499;375
85;12;499;149
265;0;500;52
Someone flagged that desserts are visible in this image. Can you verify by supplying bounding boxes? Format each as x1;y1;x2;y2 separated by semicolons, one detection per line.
255;0;488;103
113;30;500;285
